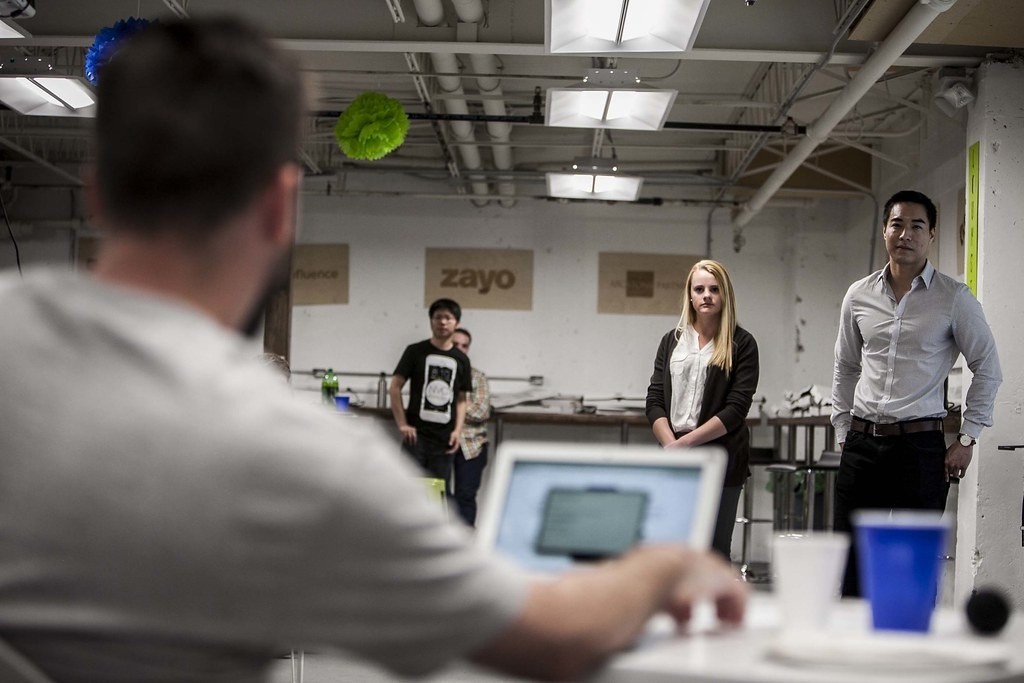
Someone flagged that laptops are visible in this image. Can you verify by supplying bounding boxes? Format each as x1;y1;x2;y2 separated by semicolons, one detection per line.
475;442;730;578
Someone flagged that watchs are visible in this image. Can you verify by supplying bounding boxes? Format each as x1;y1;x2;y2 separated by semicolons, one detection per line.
956;433;977;447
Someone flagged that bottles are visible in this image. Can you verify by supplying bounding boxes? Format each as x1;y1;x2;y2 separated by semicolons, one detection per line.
376;372;387;409
321;368;339;409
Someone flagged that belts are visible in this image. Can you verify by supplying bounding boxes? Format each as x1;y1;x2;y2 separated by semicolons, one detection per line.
850;419;943;436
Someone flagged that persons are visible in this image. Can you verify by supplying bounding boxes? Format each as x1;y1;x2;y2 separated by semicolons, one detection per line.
452;327;489;533
829;191;1002;610
391;298;474;506
646;259;760;566
1;12;747;683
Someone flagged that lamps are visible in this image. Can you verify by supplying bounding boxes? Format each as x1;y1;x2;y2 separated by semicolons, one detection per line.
932;66;975;119
546;68;678;134
0;56;100;118
544;155;643;202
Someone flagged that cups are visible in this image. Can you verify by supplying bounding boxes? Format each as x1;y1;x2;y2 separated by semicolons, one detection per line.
765;529;849;634
854;512;949;635
333;396;350;412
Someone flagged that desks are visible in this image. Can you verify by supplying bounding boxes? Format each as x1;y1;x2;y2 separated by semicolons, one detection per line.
360;408;836;551
